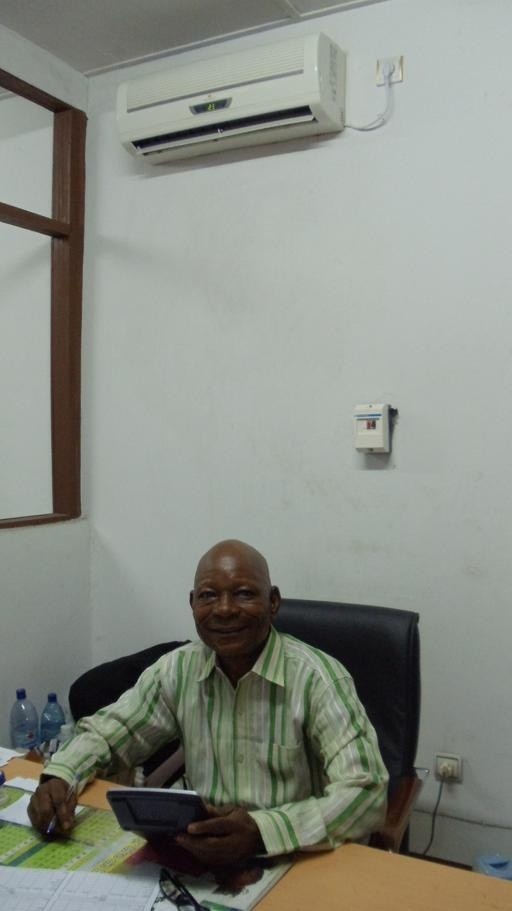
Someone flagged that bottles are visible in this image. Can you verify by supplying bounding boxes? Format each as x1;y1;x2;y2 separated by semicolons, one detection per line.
39;692;66;744
9;688;39;751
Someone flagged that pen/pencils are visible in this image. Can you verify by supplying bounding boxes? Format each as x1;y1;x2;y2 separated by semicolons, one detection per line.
43;767;82;833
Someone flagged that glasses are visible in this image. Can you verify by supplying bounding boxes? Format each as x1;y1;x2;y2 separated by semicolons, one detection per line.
160;869;209;910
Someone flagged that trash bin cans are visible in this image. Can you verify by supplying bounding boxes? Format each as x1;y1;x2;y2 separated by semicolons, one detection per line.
473;854;512;882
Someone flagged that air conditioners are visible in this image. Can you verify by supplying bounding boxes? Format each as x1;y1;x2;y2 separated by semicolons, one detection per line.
116;32;347;170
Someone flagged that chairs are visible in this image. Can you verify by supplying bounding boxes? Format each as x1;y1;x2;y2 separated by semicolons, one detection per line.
272;599;423;859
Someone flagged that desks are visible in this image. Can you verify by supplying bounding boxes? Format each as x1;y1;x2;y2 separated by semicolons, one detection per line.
1;744;511;909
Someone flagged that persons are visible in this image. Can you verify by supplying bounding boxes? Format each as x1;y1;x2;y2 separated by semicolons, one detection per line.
26;537;390;871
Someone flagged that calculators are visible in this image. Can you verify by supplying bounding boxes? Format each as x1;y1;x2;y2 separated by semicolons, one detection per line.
106;788;210;843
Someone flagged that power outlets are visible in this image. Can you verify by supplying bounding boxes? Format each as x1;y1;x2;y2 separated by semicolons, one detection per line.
432;751;462;784
